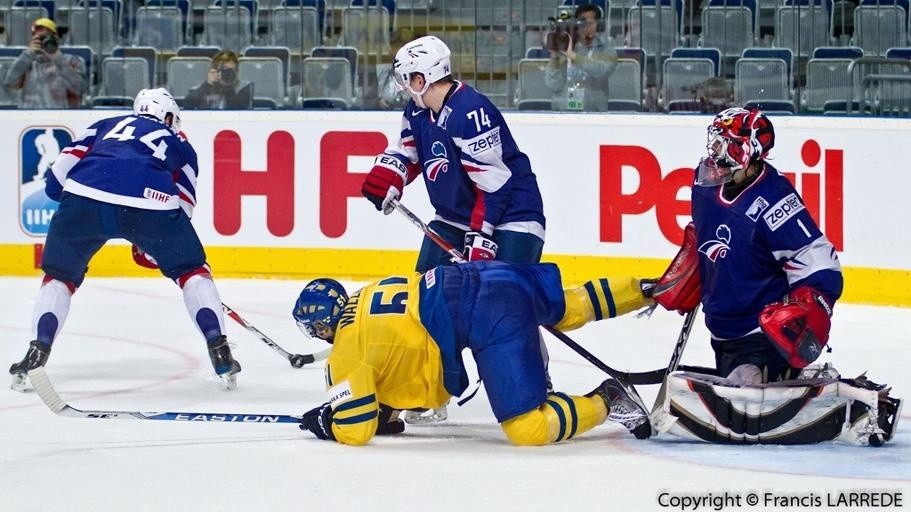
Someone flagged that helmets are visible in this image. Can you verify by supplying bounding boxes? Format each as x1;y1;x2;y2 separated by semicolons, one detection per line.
698;108;775;187
391;36;453;96
133;88;180;136
293;278;349;340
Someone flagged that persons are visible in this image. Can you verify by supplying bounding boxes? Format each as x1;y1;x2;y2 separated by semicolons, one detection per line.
5;18;86;110
8;87;242;390
652;107;904;449
362;39;412;111
363;36;545;423
546;3;619;112
183;48;254;110
292;260;663;447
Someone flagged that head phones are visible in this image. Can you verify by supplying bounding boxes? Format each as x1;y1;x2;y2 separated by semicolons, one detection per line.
594;4;605;32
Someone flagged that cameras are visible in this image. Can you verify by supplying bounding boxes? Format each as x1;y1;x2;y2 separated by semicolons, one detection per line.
216;66;237;84
35;34;59;54
543;4;587;50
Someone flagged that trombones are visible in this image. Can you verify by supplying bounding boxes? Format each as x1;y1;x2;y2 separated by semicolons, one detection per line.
221;302;333;370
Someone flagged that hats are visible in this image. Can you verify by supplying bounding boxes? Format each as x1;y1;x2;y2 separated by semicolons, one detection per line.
31;18;58;38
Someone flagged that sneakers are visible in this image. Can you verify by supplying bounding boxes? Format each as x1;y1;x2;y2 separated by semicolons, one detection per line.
584;376;650;441
8;341;51;377
207;335;241;380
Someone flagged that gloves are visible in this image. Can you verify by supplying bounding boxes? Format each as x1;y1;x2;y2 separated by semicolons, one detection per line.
652;222;702;315
299;402;337;441
361;152;410;217
450;231;499;265
760;285;833;369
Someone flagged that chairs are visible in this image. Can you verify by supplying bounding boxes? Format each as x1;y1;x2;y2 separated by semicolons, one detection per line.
880;49;911;93
166;46;222;99
92;97;133;110
271;0;326;54
824;100;874;117
608;47;647;102
175;97;189;109
807;46;865;109
854;0;907;53
136;0;192;52
237;46;290;105
628;0;684;54
4;0;55;45
341;0;396;55
204;0;259;52
555;0;611;45
776;0;834;52
302;46;359;105
0;44;28;102
252;98;277;112
302;99;347;112
518;47;551;97
607;99;642;113
669;100;722;116
103;46;157;99
59;46;94;97
69;0;124;51
518;99;552;111
735;48;793;106
743;100;797;116
701;0;757;55
663;48;721;102
